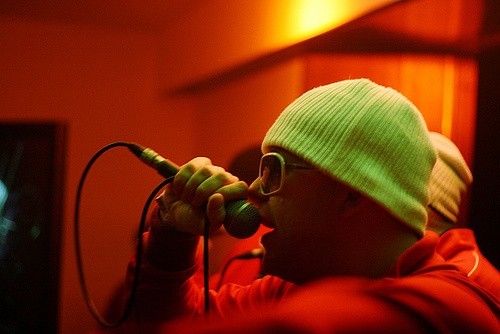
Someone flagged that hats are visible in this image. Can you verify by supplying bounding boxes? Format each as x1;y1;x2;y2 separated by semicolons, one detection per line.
427;131;472;223
260;78;437;241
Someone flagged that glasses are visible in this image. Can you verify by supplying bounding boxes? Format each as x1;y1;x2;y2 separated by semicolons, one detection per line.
260;151;313;195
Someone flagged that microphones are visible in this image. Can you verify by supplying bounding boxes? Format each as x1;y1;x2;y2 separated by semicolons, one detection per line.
128;144;260;238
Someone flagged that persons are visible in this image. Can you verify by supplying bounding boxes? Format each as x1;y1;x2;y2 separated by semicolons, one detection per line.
109;77;499;334
192;143;266;292
420;129;500;309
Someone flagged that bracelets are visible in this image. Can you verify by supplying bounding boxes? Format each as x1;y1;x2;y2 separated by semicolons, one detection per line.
155;188;198;242
